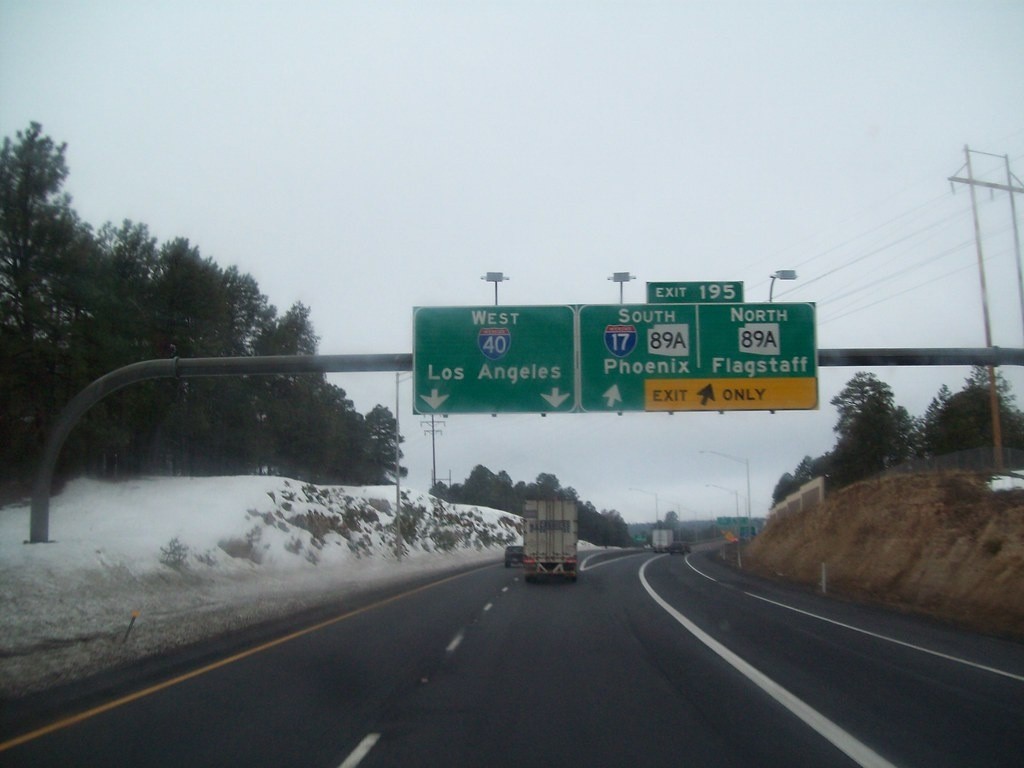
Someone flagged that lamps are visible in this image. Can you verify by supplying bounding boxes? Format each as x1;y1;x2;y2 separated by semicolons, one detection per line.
607;272;636;304
480;271;511;305
768;268;798;303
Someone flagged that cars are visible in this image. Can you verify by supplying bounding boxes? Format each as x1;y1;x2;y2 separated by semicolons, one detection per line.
670;541;690;555
505;546;524;567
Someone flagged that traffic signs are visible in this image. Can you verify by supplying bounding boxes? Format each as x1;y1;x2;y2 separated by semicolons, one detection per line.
413;303;820;412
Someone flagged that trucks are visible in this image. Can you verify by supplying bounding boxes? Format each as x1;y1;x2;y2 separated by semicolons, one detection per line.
522;500;578;583
651;529;673;553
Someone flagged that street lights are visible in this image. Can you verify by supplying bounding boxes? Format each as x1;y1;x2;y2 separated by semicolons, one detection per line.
698;449;751;544
705;484;742;569
